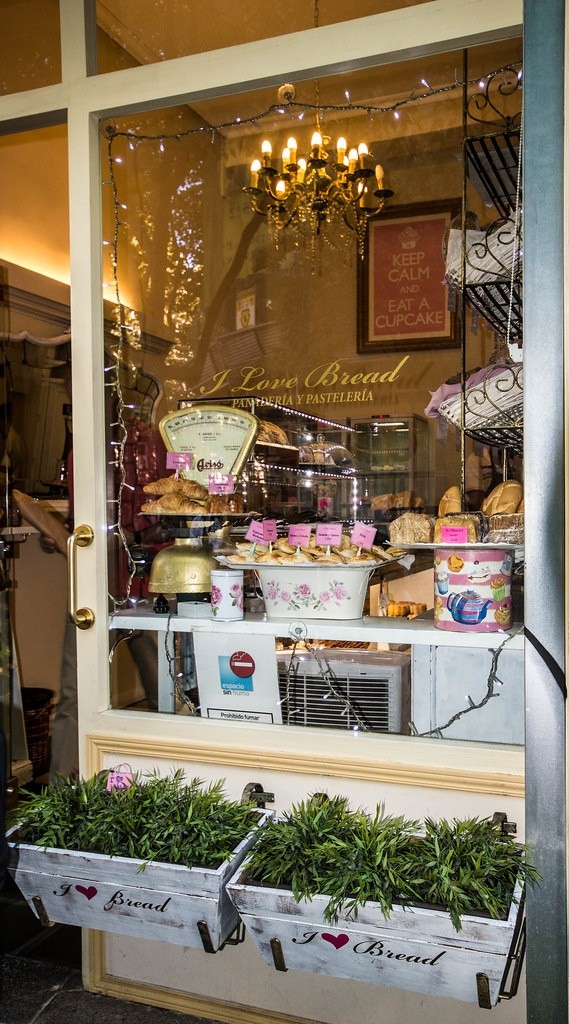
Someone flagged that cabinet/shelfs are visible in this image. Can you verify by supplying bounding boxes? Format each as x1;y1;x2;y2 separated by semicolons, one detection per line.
460;36;524;513
178;395;356;537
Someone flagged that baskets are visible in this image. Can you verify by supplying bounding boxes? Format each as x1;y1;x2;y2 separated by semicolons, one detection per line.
444;210;522;340
23;687;56;777
436;365;523;446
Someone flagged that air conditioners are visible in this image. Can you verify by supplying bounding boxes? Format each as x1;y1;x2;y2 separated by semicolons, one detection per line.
276;648;411;734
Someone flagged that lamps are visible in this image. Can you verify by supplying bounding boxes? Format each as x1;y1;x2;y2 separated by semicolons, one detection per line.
242;0;394;277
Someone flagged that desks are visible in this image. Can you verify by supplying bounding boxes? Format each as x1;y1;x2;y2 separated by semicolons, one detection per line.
110;605;524;747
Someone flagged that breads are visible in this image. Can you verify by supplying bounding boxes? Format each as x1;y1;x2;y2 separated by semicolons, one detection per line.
142;417;478;617
481;481;525;516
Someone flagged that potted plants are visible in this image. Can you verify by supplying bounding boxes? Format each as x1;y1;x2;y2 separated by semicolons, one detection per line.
5;769;275;955
225;814;526;1010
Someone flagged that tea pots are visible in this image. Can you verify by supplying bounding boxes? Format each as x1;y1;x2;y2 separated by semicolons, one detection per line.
445;589;492;625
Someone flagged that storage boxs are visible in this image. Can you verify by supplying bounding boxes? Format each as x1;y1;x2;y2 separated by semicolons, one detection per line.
256;570;372;620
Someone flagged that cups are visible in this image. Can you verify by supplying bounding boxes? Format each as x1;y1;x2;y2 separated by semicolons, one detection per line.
437;580;450;594
211;569;245;622
491;585;506;602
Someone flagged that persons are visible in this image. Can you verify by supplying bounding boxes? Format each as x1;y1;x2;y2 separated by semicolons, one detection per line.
38;361;179;796
